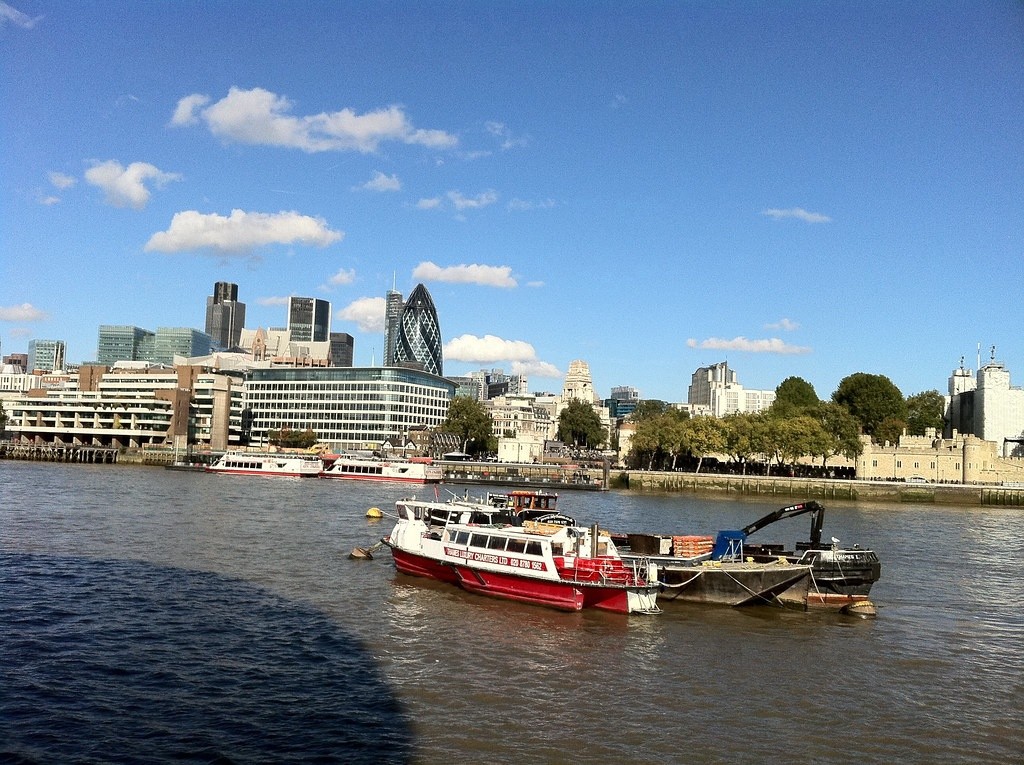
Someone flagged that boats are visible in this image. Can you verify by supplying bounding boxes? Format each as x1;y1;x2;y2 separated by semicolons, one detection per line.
610;500;879;616
380;486;663;618
